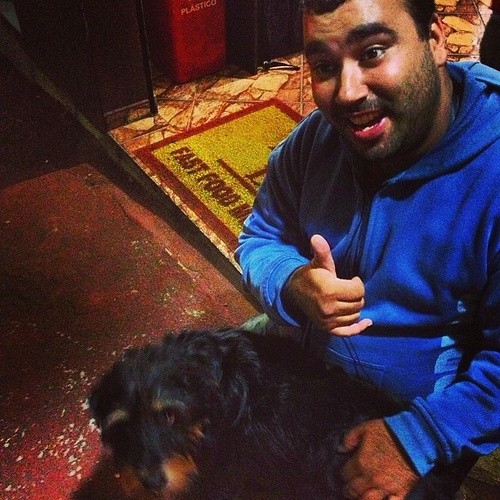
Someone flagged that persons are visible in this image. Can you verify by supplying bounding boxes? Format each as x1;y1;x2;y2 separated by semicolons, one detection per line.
234;0;500;500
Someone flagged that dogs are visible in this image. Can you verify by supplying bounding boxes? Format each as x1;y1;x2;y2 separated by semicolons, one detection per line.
83;322;453;500
